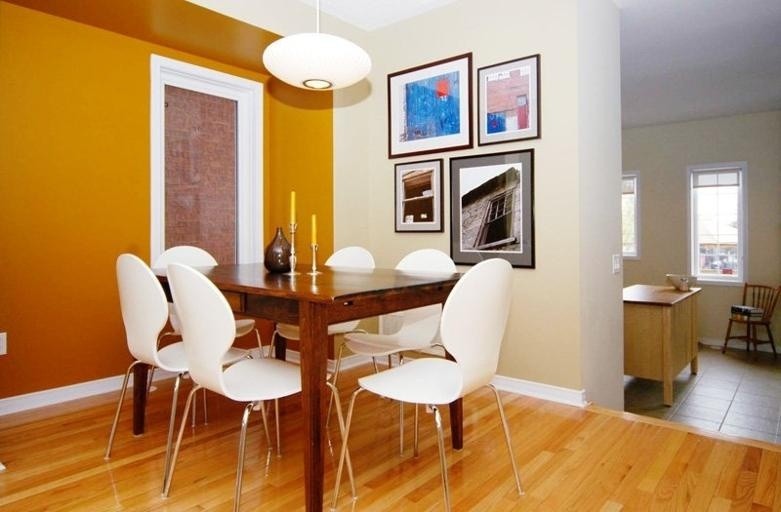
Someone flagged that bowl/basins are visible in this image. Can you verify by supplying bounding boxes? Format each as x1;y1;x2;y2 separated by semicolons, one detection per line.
666;273;697;291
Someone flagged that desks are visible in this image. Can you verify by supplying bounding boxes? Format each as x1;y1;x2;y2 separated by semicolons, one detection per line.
623;284;702;407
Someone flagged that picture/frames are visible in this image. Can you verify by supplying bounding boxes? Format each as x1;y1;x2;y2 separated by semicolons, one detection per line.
387;52;474;158
449;148;535;271
478;53;541;146
394;157;444;233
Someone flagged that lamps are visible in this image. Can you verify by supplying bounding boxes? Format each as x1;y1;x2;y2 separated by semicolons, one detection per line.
262;32;371;91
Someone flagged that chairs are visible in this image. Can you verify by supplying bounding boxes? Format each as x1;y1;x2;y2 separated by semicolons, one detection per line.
266;246;376;428
147;245;265;428
722;282;781;364
103;253;272;496
331;258;524;512
325;248;457;457
164;263;357;512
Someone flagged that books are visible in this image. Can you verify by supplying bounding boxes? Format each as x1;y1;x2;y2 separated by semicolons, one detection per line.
730;304;765;322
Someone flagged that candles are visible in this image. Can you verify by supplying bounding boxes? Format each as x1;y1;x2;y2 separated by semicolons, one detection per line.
290;190;296;224
311;214;317;244
